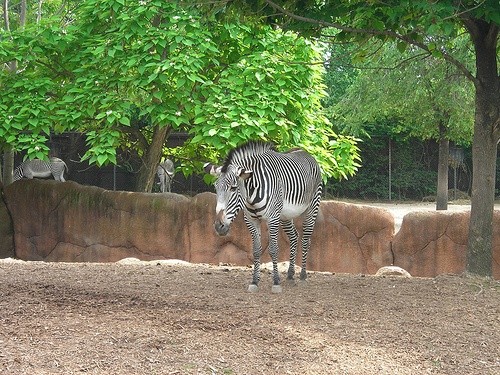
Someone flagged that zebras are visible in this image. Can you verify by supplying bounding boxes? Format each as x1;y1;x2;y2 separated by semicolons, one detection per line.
12;157;69;183
156;156;175;193
203;138;324;294
